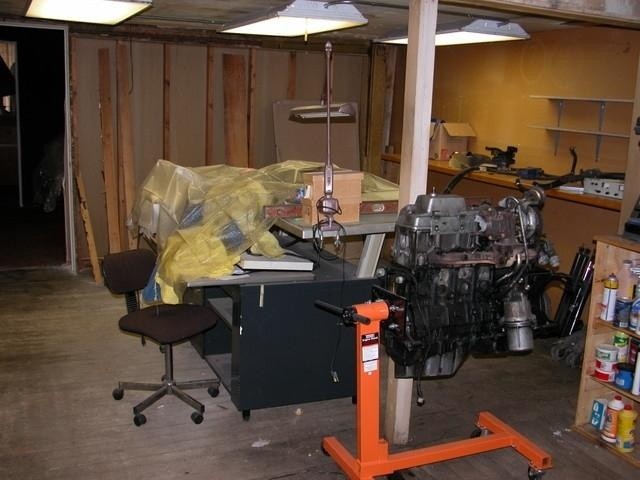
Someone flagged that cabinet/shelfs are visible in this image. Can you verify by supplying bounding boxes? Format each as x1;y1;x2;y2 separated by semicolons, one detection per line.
525;95;634;162
570;235;640;469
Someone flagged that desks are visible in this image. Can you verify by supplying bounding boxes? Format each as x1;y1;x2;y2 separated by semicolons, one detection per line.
274;215;414;279
131;221;378;421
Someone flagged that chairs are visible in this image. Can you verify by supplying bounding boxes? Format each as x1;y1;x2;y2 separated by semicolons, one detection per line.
102;248;219;427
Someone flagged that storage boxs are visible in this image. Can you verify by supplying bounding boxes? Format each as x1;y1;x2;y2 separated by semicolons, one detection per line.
429;123;476;161
295;168;364;223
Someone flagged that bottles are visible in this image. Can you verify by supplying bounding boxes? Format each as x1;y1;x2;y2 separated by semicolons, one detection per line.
600;395;624;443
600;273;618;321
613;332;640;395
618;260;635;301
616;405;636;453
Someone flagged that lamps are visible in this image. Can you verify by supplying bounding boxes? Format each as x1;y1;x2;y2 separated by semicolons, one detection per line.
216;0;368;47
373;18;531;46
24;0;153;26
292;40;355;229
